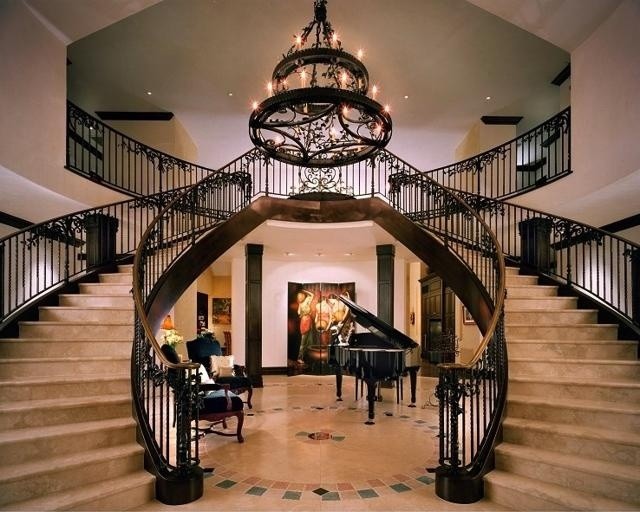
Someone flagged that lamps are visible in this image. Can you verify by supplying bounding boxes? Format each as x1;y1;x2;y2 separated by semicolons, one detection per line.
247;1;394;170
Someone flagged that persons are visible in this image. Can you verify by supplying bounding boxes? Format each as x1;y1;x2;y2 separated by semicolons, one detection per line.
328;293;348;321
295;288;315;365
315;294;332;342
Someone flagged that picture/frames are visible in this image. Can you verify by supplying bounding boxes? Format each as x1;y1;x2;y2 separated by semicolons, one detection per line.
462;306;478;326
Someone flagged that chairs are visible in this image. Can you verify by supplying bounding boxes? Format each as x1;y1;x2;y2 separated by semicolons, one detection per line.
161;332;254;444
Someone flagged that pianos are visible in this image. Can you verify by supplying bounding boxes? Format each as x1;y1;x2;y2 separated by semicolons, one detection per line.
328;294;419;424
